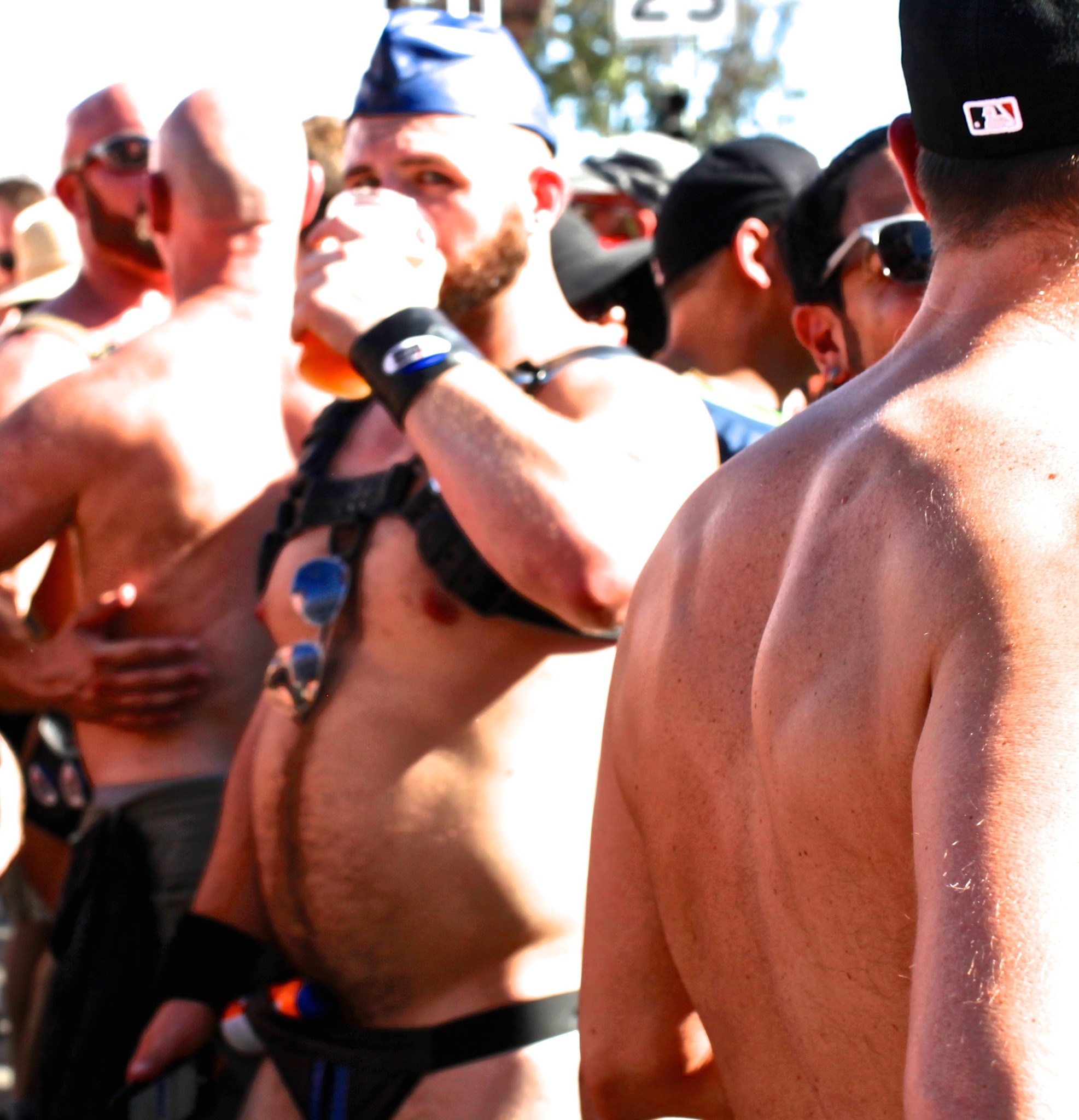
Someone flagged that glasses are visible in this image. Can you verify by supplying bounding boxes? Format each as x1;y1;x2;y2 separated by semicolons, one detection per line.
817;214;935;296
75;130;152;176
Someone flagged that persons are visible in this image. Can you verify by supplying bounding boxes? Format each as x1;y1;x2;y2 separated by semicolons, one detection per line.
0;0;1079;1120
127;8;724;1120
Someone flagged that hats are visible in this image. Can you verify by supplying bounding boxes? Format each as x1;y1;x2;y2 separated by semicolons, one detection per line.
657;137;821;276
550;192;653;304
352;8;554;155
901;3;1076;155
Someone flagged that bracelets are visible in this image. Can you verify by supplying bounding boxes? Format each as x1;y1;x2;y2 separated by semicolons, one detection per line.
159;900;274;1015
342;308;481;424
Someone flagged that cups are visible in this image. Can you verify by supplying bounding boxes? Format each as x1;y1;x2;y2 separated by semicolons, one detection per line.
294;188;437;401
